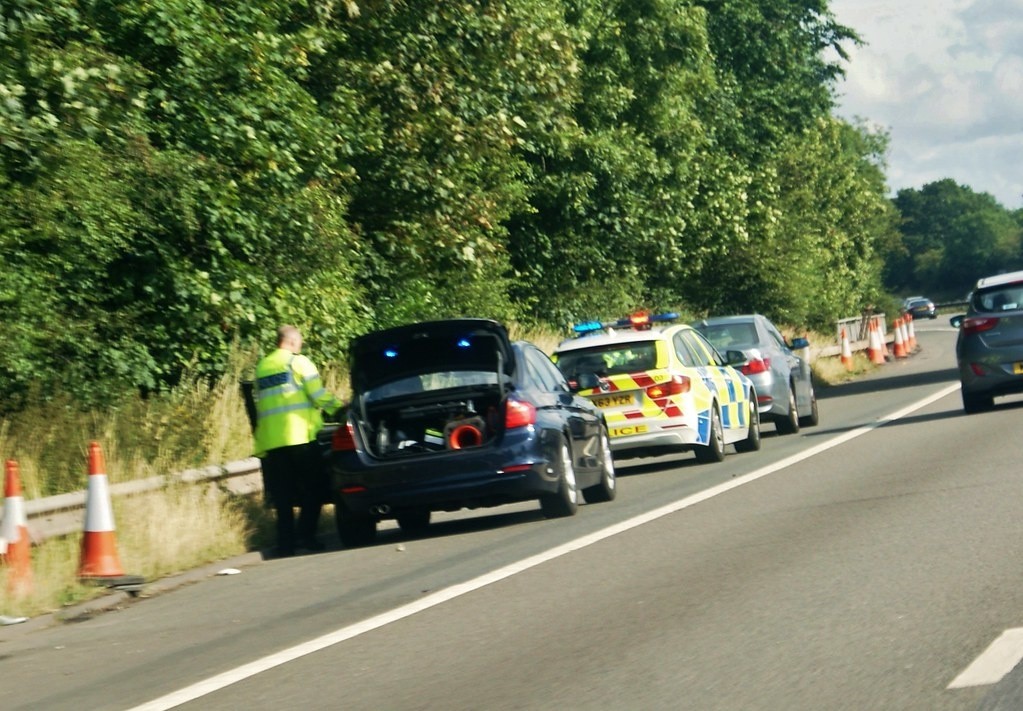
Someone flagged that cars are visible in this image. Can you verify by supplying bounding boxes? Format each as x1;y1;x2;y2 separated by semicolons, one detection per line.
550;312;761;462
904;296;938;320
686;313;819;435
948;271;1023;413
238;319;617;549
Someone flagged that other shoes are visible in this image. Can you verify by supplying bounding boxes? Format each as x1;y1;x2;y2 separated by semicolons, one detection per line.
281;546;294;556
289;535;325;550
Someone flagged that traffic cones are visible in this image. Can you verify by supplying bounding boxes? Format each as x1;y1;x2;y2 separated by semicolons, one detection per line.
1;457;50;608
839;328;855;373
868;317;891;365
74;441;146;586
892;314;921;360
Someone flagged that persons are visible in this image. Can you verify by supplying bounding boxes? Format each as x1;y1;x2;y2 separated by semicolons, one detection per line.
250;325;343;554
